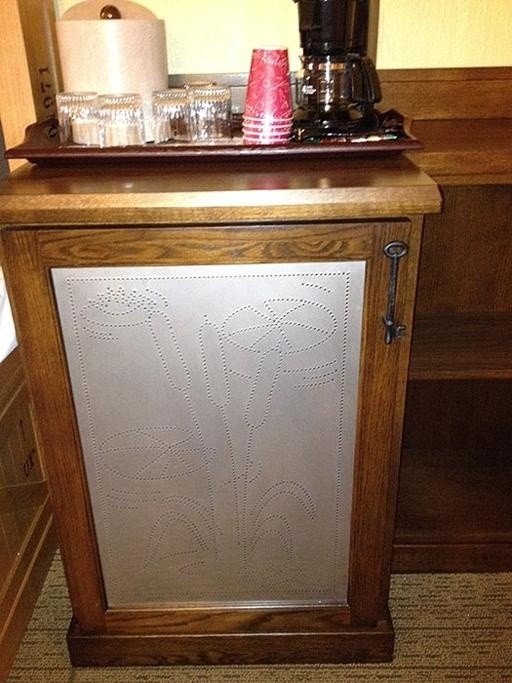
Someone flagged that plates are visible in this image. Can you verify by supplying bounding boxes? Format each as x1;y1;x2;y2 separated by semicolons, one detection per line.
4;111;427;166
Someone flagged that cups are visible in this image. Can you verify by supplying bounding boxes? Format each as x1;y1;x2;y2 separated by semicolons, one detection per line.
182;79;213;88
55;90;97;99
57;101;102;148
102;102;144;146
192;86;233;138
240;48;295;145
150;88;193;96
96;93;141;102
152;96;194;148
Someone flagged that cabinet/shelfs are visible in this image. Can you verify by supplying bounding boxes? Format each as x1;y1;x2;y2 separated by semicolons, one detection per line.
386;118;512;575
0;152;441;665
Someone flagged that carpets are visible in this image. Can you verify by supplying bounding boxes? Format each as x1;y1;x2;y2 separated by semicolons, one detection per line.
1;549;512;683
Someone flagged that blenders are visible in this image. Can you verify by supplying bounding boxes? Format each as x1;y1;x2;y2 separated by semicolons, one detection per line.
294;0;384;143
